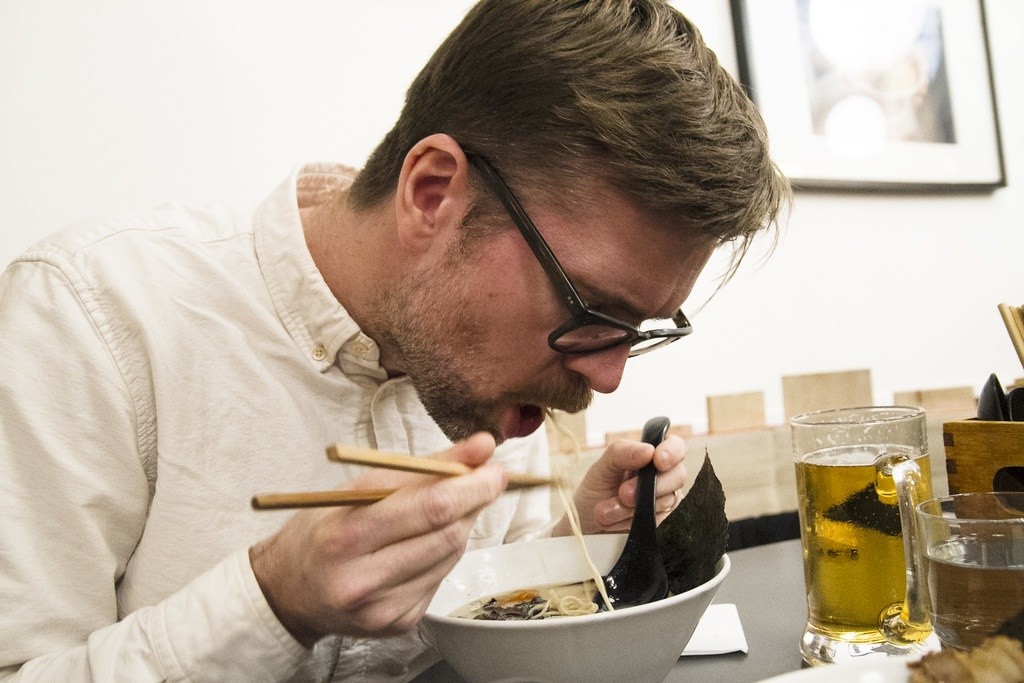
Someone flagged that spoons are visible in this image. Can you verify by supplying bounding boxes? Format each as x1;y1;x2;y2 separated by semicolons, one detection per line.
977;373;1024;512
593;417;668;612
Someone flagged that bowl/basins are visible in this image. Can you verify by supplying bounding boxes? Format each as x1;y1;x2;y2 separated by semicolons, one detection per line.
417;534;731;683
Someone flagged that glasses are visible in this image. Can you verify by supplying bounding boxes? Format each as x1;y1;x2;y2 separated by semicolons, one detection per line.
462;146;693;359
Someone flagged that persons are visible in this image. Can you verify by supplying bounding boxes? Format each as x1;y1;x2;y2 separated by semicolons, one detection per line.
1;0;793;681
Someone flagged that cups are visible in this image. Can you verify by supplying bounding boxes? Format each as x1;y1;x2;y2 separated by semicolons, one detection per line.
789;406;932;665
915;491;1024;653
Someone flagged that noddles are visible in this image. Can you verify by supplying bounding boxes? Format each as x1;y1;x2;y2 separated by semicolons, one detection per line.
465;411;674;621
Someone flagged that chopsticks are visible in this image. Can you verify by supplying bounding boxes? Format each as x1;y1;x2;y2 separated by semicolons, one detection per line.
252;443;560;509
998;304;1024;371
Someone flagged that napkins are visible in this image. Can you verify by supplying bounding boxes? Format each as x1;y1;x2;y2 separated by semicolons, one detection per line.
679;602;750;658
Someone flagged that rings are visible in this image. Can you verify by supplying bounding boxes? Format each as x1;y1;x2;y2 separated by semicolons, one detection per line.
665;490;678;512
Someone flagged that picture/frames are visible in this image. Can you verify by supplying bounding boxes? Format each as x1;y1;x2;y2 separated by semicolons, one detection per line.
729;0;1008;195
